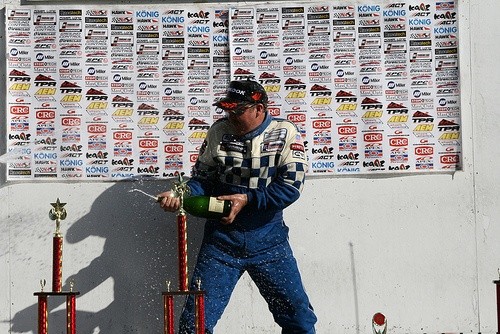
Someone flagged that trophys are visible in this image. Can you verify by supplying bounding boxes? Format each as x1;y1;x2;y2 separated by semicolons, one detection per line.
33;195;80;332
159;174;207;334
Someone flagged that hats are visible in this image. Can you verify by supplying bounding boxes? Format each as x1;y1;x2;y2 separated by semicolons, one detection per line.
212;80;268;115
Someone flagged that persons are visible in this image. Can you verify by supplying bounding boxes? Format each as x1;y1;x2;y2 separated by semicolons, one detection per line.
156;80;317;334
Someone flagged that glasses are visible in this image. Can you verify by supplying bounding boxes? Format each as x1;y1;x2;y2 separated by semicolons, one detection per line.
228;103;258;115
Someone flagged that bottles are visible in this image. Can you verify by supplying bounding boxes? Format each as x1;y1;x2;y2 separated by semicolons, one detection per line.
155;195;232;218
